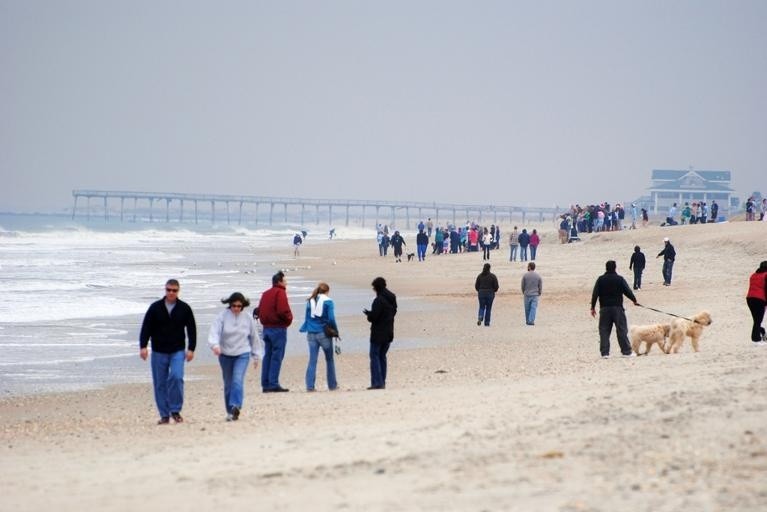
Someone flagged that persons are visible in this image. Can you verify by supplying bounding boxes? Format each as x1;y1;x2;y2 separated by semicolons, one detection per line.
301;230;306;239
299;282;341;393
139;280;197;425
329;228;337;239
256;271;293;392
293;234;302;257
363;277;397;390
207;293;260;422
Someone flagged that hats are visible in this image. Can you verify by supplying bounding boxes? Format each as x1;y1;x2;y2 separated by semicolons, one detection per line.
664;238;669;241
373;277;386;288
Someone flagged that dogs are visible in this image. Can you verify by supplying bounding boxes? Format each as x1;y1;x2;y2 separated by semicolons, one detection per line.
665;310;714;354
408;253;414;262
629;323;671;356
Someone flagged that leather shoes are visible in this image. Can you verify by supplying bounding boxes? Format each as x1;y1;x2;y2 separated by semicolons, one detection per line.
263;388;288;392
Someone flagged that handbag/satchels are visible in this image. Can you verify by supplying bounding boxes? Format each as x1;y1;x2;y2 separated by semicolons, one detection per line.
324;323;336;337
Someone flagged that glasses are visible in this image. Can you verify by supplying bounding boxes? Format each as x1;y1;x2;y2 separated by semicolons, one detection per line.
166;287;179;292
231;304;242;308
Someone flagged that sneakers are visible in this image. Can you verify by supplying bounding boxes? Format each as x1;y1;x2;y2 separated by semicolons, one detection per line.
232;407;239;420
158;418;169;424
227;414;233;421
172;411;182;422
